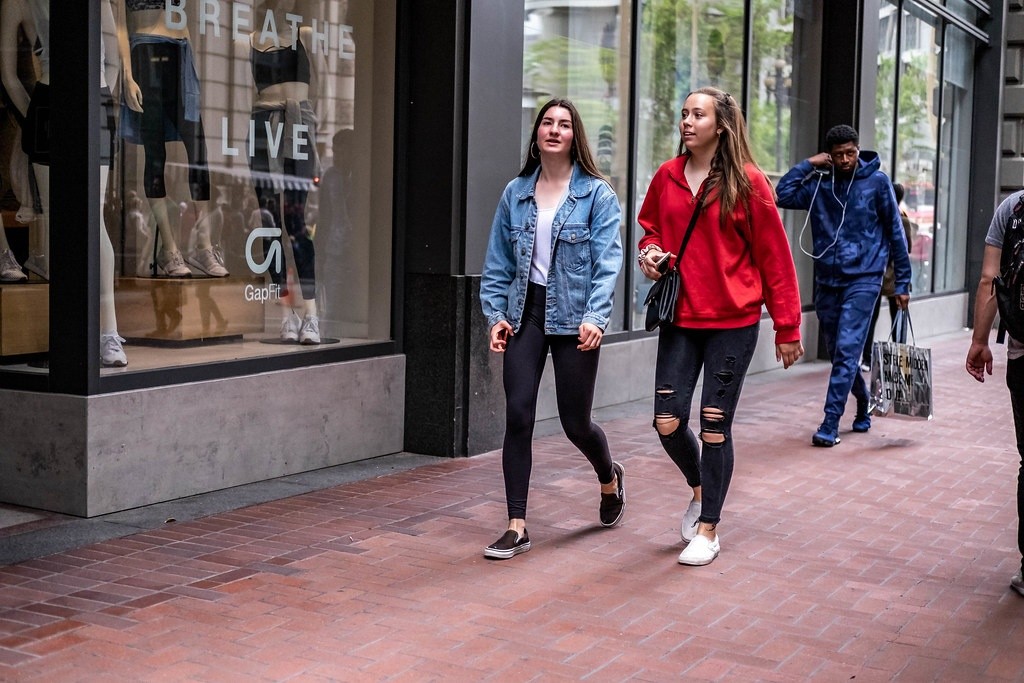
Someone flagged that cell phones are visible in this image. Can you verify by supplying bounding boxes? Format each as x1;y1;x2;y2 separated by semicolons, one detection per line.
654;252;672;281
501;329;510;349
815;165;830;175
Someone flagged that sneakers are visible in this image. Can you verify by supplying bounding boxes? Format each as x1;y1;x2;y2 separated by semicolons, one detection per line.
852;410;870;432
677;531;720;566
187;246;230;279
100;333;127;366
299;313;321;343
681;497;705;543
156;247;193;279
24;254;51;280
0;248;27;281
812;425;840;445
280;310;304;343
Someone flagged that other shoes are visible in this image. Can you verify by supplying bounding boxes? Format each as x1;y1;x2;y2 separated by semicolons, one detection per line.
1011;570;1024;595
599;460;626;527
861;361;872;372
484;527;531;559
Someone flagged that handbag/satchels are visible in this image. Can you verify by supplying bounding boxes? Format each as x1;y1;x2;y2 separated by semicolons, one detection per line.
643;269;681;331
882;264;895;296
866;305;932;420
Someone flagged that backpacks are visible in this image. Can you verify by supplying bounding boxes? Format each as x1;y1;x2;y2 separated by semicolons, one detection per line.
990;193;1024;345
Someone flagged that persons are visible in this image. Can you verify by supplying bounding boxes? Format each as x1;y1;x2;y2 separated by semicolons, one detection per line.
966;189;1024;594
479;98;625;558
638;88;804;565
776;126;912;445
245;0;332;343
314;127;353;344
119;0;229;277
105;182;275;342
0;0;128;368
860;184;912;371
